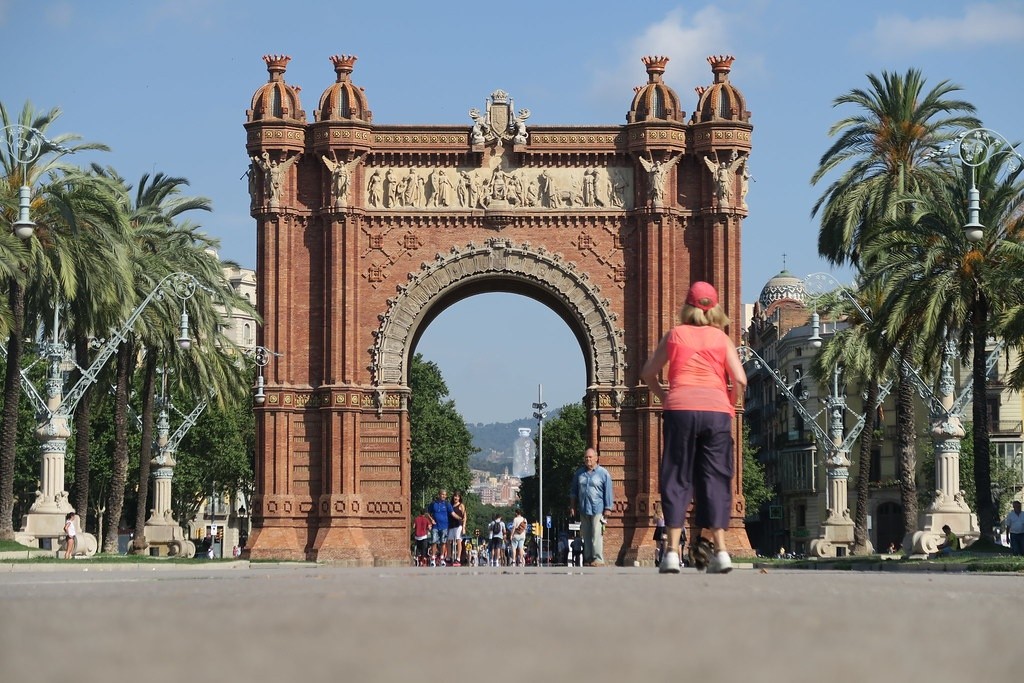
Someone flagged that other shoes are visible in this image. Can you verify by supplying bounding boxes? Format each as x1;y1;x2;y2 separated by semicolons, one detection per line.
452;559;461;566
439;558;447;566
518;562;523;566
496;561;500;567
491;561;494;565
679;561;684;568
430;559;436;567
655;559;659;567
591;561;605;567
511;561;516;566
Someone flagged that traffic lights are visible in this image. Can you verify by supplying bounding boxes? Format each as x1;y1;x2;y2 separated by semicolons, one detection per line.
219;529;223;538
215;533;218;539
532;522;539;535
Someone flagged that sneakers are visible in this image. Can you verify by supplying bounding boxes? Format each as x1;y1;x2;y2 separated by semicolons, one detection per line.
659;553;681;573
706;551;733;573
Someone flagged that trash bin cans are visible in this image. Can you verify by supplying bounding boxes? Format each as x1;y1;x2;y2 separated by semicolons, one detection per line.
204;537;221;559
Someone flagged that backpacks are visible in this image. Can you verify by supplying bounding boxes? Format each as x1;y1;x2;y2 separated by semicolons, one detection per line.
493;520;502;534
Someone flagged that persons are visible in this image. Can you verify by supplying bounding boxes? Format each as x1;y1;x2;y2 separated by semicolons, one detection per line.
64;512;76;560
937;525;957;558
642;281;747;575
570;447;613;567
1006;501;1024;557
888;542;895;556
413;487;689;567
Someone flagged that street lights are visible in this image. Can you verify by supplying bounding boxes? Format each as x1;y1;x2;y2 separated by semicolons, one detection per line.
532;402;548;567
237;505;246;554
22;271;196;550
801;271;982;555
145;346;271;559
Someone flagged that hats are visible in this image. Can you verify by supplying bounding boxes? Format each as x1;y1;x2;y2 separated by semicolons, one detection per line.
685;281;719;309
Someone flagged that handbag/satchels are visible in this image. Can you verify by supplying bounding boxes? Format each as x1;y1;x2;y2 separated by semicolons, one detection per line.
516;521;527;534
